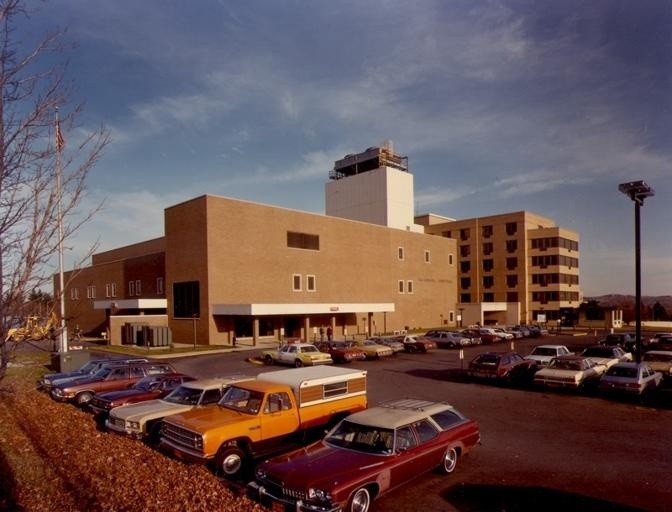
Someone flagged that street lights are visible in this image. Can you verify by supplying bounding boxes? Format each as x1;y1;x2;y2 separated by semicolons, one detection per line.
619;179;656;367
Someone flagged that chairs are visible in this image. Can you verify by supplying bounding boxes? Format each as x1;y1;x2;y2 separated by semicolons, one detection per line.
353;430;410;451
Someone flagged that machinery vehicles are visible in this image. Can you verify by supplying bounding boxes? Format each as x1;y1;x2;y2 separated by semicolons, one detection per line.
7;309;59;343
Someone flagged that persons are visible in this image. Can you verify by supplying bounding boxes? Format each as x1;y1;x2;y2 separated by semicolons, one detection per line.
319;323;325;342
327;324;332;341
71;321;84;343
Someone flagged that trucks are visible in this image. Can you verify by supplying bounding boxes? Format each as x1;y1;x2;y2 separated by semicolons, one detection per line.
158;365;369;479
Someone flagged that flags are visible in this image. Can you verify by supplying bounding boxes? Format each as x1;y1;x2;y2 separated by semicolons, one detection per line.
55;121;66;153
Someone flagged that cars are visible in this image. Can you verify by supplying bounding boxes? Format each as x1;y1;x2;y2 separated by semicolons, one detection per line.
40;357;198;415
263;322;548;365
104;374;259;440
247;396;480;511
466;332;672;402
